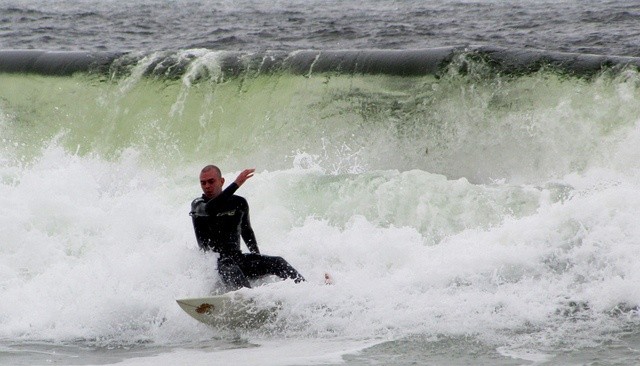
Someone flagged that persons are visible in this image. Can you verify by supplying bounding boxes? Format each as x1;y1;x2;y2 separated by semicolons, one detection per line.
191;164;307;291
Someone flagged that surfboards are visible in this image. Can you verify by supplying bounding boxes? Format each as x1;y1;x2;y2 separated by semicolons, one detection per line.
176;270;332;333
